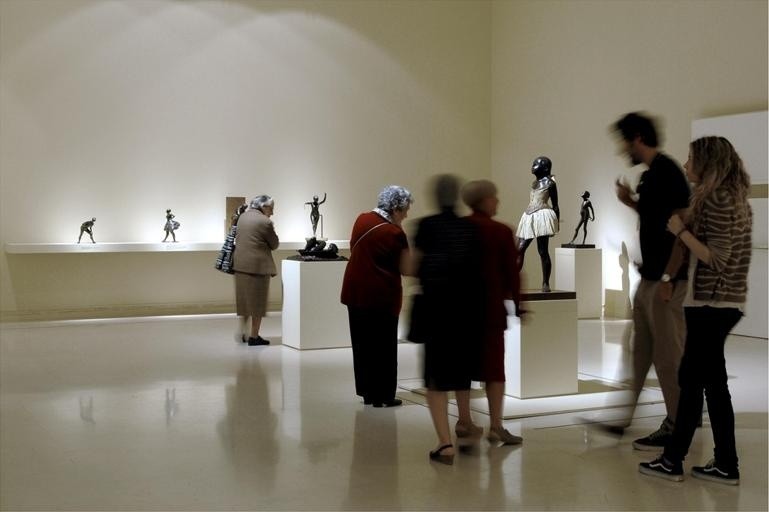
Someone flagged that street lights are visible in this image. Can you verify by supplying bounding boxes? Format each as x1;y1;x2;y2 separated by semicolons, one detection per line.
215;234;236;274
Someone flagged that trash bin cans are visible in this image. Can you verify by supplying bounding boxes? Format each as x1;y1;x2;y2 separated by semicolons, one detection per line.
660;272;674;284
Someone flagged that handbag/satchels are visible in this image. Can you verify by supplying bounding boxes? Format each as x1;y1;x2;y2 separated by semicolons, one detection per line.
675;227;689;239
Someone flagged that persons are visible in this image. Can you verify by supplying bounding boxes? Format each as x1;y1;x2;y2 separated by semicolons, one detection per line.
77;217;97;243
514;156;560;292
231;194;280;345
302;191;327;235
339;184;424;408
575;110;704;454
406;170;485;466
228;203;248;231
161;208;177;241
454;179;536;445
637;135;754;486
569;190;595;244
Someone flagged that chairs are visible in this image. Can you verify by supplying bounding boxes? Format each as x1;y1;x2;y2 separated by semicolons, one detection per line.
632;428;666;452
489;428;523;442
691;458;740;486
639;454;684;481
455;421;484;438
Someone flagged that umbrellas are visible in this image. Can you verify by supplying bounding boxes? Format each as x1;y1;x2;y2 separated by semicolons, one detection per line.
373;398;403;408
248;336;271;346
363;397;373;405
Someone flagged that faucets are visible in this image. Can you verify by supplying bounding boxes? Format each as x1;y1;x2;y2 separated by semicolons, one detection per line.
429;443;455;465
